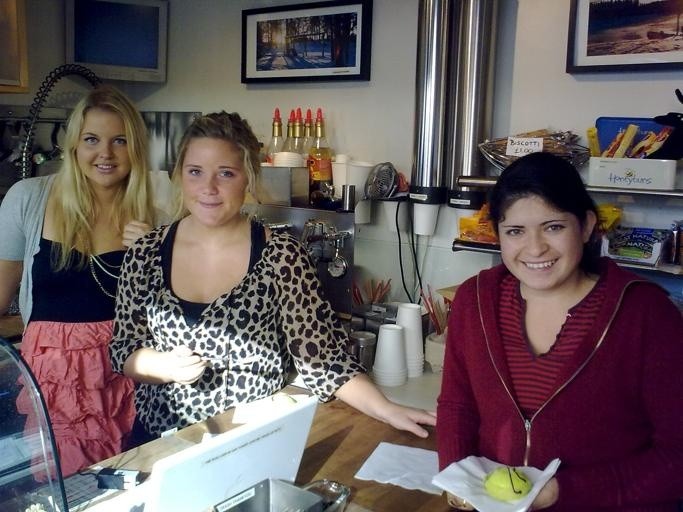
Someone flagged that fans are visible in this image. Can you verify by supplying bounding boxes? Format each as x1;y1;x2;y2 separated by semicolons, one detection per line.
364;162;399;201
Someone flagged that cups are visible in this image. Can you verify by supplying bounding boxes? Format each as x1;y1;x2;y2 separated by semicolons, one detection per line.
413;202;440;236
370;301;424;387
348;331;377;371
385;199;408;235
347;159;373;198
300;217;337;260
330;154;350;199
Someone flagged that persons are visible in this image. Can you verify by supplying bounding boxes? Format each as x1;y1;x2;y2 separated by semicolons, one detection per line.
435;152;683;512
1;82;152;482
110;109;438;451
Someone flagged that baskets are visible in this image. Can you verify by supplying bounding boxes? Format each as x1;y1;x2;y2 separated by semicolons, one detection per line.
478;137;592;172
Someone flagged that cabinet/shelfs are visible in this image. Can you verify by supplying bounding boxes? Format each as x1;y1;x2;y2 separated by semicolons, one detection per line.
451;176;683;272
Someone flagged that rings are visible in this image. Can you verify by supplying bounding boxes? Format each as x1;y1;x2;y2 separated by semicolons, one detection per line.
463;499;467;508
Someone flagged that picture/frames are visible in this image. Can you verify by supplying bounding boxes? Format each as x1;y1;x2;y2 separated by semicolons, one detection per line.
566;0;683;73
0;0;31;93
241;0;373;83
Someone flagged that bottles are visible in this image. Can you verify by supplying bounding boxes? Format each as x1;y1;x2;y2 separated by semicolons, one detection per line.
265;105;337;206
